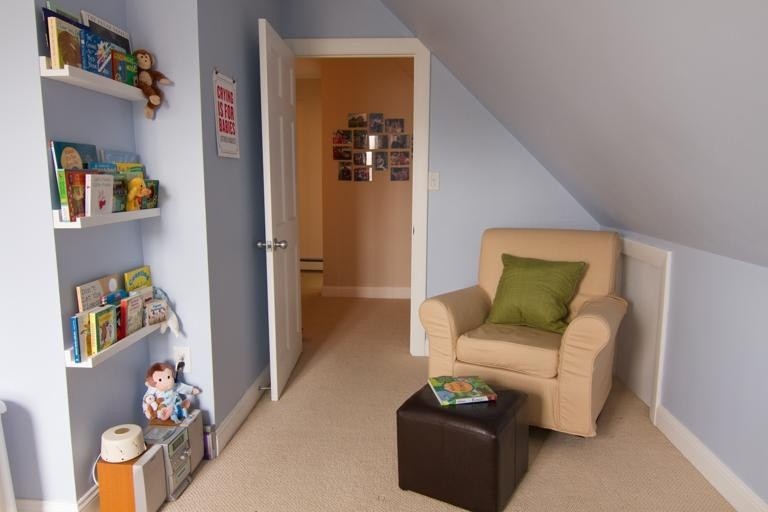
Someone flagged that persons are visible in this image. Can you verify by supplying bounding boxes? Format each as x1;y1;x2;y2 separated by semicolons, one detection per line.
332;114;415;183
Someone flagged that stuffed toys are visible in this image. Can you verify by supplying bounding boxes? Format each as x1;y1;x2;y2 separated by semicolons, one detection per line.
139;358;200;424
131;49;175;120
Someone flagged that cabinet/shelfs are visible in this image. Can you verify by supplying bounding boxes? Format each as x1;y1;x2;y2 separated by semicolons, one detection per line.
39;56;160;369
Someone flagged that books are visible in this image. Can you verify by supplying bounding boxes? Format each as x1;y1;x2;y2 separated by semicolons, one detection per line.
39;0;140;87
428;373;496;407
50;141;169;366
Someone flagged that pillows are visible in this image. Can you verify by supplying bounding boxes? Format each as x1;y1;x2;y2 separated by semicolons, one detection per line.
484;254;586;336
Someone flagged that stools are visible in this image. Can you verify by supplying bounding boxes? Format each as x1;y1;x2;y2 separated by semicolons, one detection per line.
397;380;529;511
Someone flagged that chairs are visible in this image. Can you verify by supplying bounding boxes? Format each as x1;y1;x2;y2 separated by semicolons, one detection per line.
418;227;628;437
